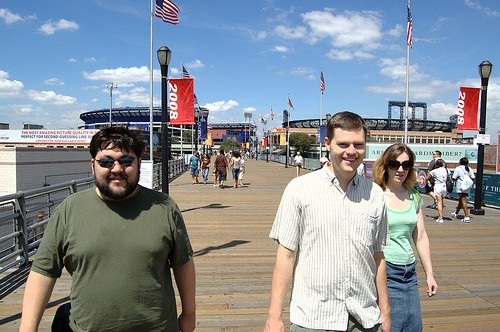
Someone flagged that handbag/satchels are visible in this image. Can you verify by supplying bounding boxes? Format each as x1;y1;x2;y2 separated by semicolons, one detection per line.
445;168;454;193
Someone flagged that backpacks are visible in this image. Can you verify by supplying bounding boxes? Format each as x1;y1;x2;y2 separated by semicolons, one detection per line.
457;166;474;192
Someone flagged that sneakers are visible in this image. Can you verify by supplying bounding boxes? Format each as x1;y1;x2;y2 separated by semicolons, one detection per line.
461;217;471;223
451;211;458;219
435;217;443;223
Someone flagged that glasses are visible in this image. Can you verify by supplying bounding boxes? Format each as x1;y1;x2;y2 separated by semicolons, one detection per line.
94;155;138;168
388;160;412;171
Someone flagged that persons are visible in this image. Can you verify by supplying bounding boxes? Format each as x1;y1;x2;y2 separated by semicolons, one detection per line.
264;112;392;332
356;162;365;177
294;152;303;177
188;151;248;188
425;150;452;223
19;127;196;332
251;152;254;159
450;158;476;223
373;141;438;332
320;157;329;168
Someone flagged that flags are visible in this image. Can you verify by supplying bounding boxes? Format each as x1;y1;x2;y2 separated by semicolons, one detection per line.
320;71;325;95
198;107;203;116
288;98;294;109
271;109;274;120
407;5;413;48
154;0;180;25
182;66;198;103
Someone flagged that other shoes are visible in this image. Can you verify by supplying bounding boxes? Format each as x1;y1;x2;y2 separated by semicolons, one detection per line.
429;202;438;210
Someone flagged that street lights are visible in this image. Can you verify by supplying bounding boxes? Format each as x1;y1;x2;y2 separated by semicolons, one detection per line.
325;113;331;161
255;137;258;160
157;45;173;194
285;126;290;168
106;81;118;126
469;60;494;215
